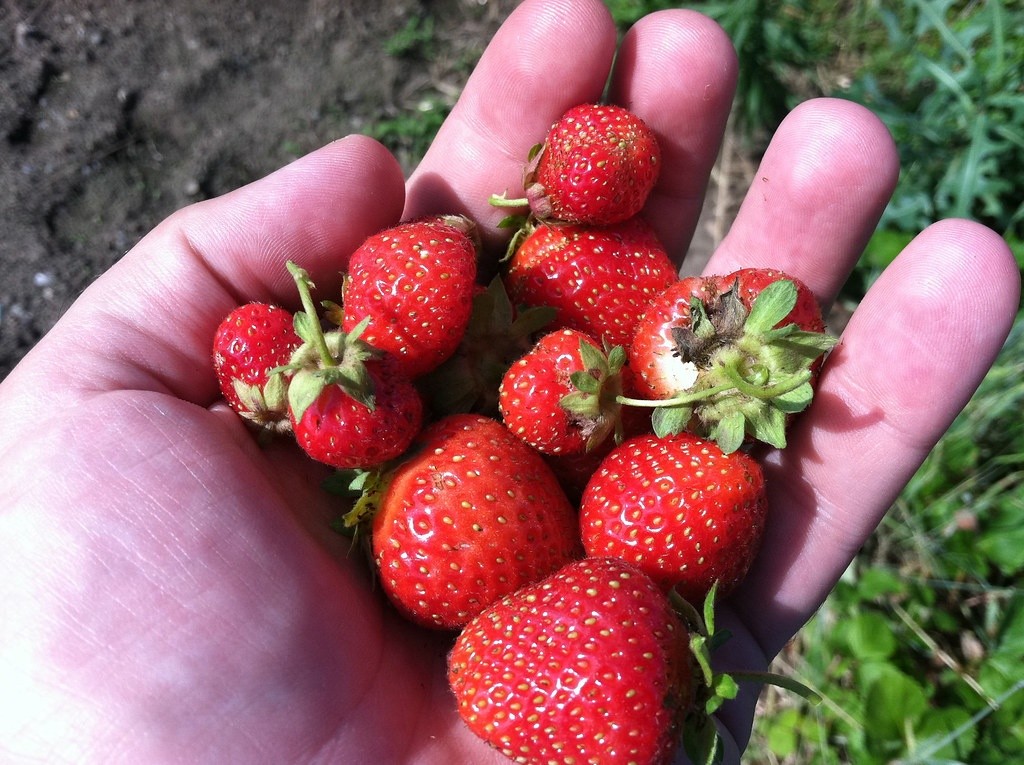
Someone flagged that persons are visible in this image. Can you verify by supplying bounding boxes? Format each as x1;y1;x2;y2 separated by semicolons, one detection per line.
0;0;1024;765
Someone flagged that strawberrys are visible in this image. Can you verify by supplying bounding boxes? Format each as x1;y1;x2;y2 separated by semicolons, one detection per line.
210;102;843;765
